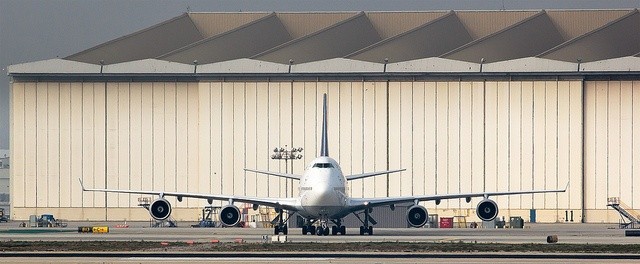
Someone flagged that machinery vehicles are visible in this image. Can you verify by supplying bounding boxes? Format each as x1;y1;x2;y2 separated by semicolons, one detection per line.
37;214;67;227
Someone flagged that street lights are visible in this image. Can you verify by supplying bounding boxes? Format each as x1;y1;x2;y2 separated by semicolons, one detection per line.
271;145;303;219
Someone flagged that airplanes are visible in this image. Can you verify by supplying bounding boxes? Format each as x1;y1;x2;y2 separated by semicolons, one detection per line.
79;93;569;234
